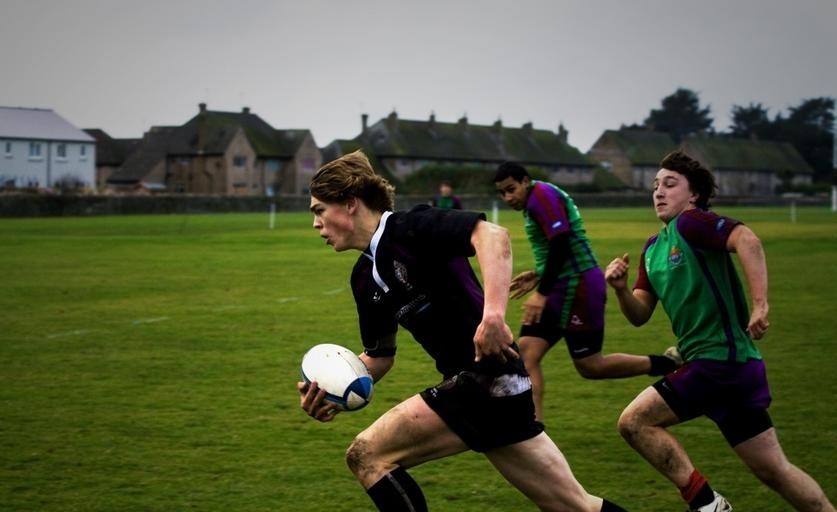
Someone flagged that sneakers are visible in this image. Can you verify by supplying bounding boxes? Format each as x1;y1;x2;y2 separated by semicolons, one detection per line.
662;346;684;367
692;490;733;512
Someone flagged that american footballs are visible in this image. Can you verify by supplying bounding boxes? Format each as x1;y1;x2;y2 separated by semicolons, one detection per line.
301;342;374;412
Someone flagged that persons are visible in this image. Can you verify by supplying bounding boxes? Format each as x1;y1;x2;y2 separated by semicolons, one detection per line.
294;148;630;512
490;160;684;429
428;176;464;211
602;148;836;512
